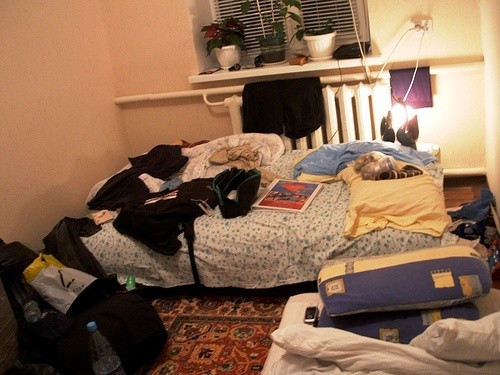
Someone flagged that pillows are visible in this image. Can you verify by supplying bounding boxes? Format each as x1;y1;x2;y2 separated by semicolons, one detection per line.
317;244;489;318
293;141;436;176
318;304;478;343
339;159;446;237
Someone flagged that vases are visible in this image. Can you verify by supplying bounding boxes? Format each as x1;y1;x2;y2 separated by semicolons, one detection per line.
201;15;247;69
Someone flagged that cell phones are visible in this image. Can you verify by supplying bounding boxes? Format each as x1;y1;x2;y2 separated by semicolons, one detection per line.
304;305;317;322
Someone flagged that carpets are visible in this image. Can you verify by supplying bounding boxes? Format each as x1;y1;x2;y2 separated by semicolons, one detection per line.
149;298;287;371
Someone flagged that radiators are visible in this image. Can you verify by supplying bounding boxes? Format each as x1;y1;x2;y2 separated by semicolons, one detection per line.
224;79;391;151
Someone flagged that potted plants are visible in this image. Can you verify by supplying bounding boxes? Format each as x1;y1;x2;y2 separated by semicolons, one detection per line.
242;0;300;64
302;21;338;61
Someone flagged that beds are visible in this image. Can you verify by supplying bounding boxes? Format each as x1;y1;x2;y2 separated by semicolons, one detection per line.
78;144;441;299
